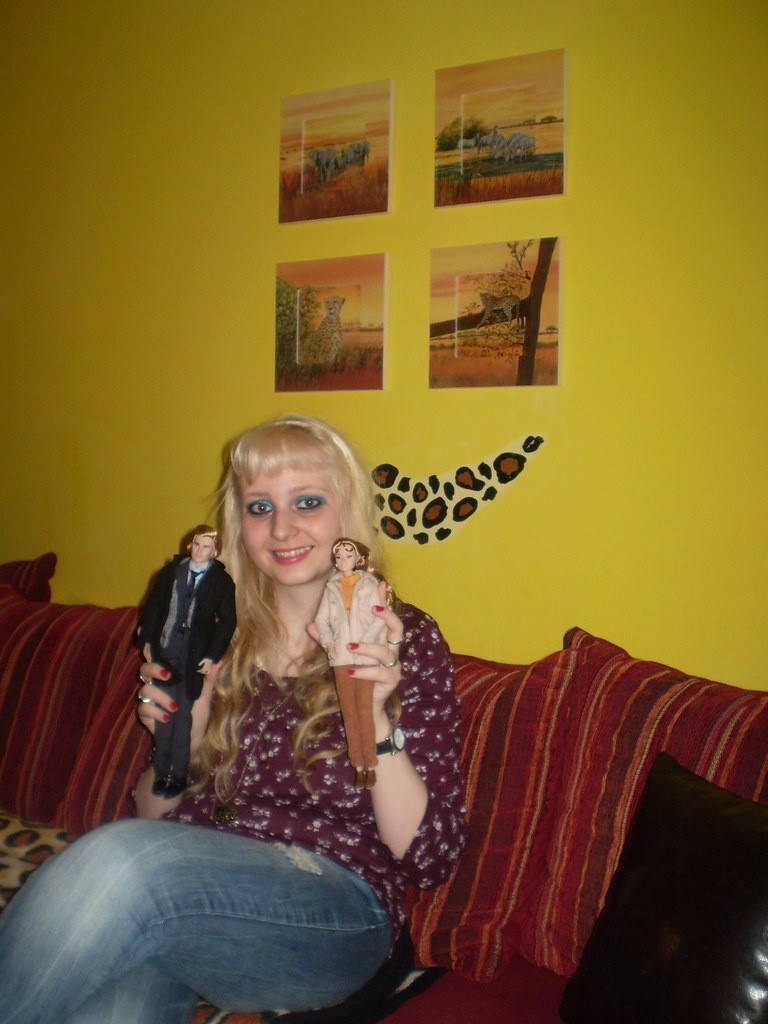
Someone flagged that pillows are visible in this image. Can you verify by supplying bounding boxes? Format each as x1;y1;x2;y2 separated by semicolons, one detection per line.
1;551;59;603
0;586;142;829
522;623;767;979
557;749;767;1023
47;640;156;844
400;645;583;986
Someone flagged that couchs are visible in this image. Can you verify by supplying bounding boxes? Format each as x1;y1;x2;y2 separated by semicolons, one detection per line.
0;551;767;1024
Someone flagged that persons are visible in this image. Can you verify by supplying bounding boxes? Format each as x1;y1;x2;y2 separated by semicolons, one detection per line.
314;537;395;790
140;525;236;800
0;415;469;1024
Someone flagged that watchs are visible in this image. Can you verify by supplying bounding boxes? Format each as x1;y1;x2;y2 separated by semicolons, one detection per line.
376;727;405;756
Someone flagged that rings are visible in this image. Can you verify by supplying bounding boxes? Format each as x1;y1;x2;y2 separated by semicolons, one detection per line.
387;637;403;645
381;657;398;669
138;674;154;685
138;690;152;703
